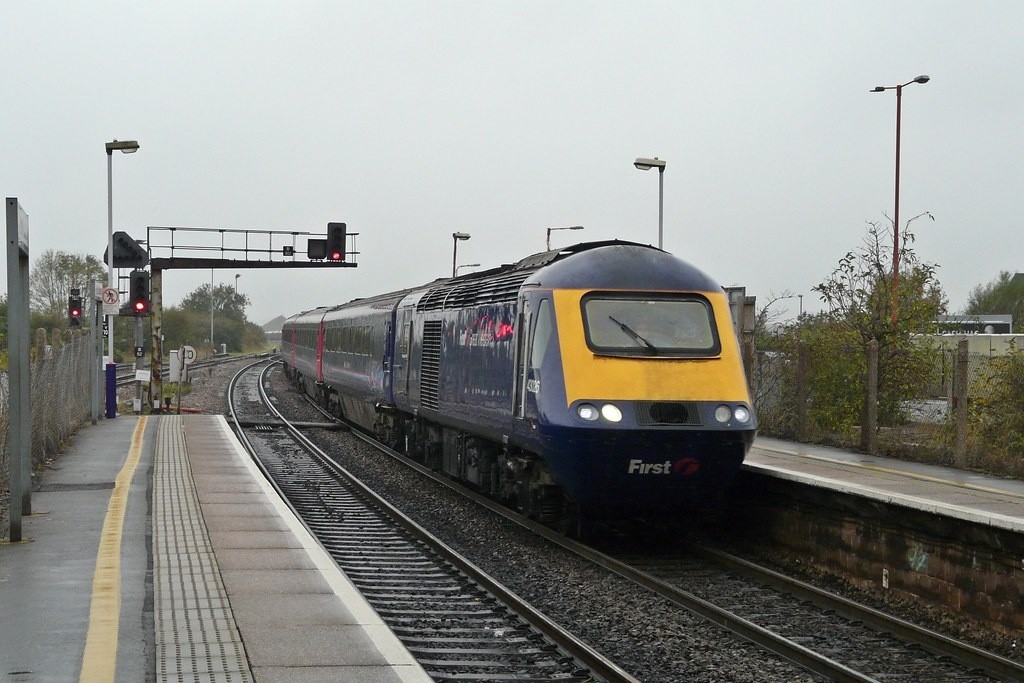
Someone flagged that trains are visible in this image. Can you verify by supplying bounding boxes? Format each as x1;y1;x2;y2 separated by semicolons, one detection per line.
281;239;759;544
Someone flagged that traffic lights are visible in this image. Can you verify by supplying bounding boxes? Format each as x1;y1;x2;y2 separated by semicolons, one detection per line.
68;296;81;325
130;269;150;313
327;221;345;260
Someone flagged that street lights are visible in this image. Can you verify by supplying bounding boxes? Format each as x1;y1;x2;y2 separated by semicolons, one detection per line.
235;274;241;295
453;231;471;278
454;264;481;278
546;226;584;252
105;141;141;421
870;74;931;337
634;156;666;252
